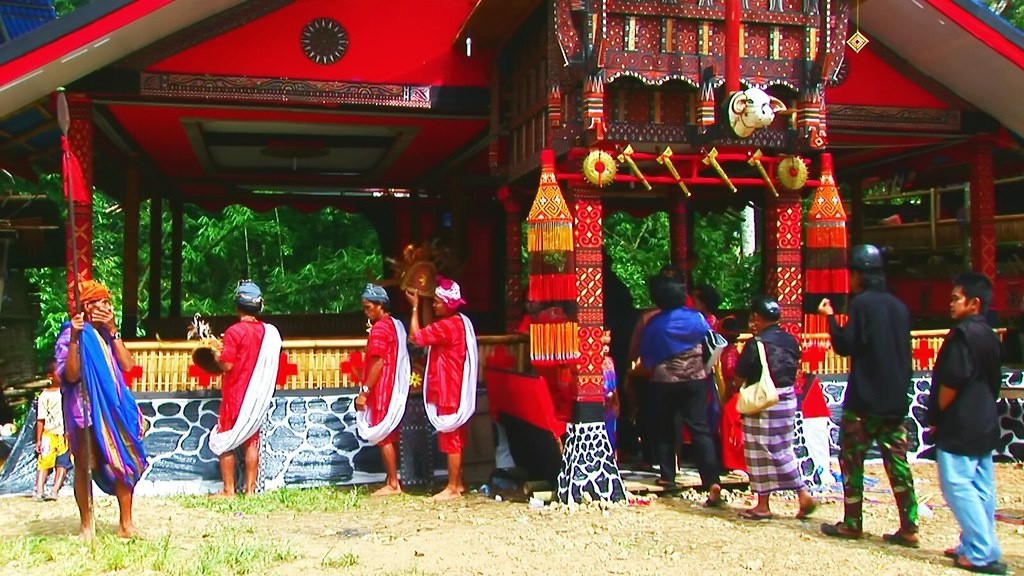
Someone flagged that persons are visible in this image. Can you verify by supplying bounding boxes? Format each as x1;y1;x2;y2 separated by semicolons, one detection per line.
726;296;817;520
208;282;282;498
817;243;920;548
35;356;74;501
0;381;14;473
517;286;570;336
54;281;148;541
687;253;699;294
928;272;1007;572
604;253;750;506
355;282;411;497
407;274;479;500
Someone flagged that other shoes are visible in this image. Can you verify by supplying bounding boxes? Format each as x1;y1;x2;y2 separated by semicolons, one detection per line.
43;492;58;501
34;495;45;503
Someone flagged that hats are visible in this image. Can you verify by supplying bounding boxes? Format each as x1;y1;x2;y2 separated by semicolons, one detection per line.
78;280;110;306
236;282;261;312
434;275;466;310
362;284;390;303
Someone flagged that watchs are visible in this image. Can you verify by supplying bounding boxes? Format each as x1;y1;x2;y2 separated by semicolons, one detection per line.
362;385;370;393
110;333;121;340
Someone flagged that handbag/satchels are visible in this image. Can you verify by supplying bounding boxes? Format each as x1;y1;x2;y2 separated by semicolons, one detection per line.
737;333;779;415
697;312;727;375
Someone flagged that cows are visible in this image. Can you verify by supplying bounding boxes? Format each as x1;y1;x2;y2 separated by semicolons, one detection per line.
728;88;786;138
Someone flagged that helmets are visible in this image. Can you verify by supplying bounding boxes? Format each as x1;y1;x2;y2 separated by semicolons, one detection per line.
847;243;883;271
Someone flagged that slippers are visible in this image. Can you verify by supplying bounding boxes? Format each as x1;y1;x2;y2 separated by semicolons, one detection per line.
882;529;919;547
945;547;958;557
795;503;814;518
954;556;996;571
655;478;676;487
738;508;772;521
821;522;861;538
703;498;725;506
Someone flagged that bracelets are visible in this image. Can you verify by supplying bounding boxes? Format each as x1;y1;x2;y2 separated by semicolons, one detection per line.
209;348;217;354
411;307;419;312
